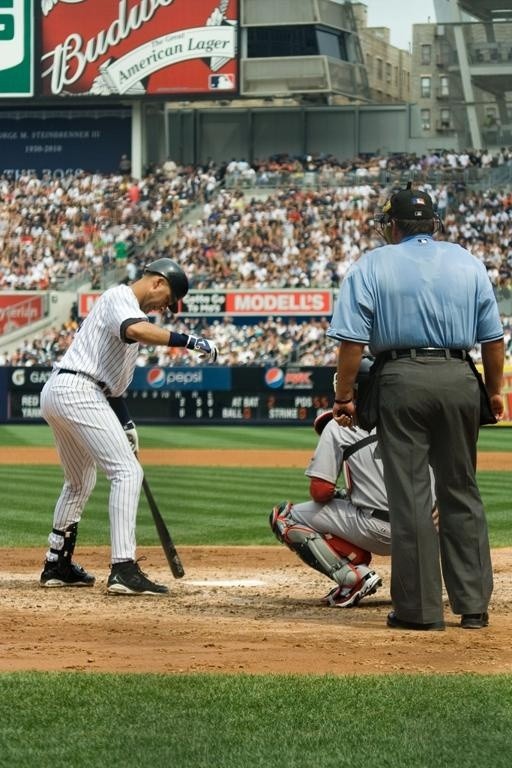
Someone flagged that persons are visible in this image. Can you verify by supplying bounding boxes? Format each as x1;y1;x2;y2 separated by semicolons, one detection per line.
38;257;219;597
268;350;441;608
1;147;512;367
331;187;506;630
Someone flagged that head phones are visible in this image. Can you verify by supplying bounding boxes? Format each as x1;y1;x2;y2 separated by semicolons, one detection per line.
373;198;440;240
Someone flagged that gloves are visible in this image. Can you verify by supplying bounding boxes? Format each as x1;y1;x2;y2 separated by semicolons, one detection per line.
123;420;138;454
186;334;218;363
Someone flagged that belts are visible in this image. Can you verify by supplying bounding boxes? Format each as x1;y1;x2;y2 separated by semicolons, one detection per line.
375;349;468;360
359;507;390;523
57;368;112;397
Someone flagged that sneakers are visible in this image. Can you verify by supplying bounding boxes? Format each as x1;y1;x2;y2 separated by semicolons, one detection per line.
320;564;382;607
40;560;95;587
461;613;489;629
386;612;445;630
105;556;168;596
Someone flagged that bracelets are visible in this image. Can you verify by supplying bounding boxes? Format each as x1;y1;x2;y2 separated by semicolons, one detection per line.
333;398;353;404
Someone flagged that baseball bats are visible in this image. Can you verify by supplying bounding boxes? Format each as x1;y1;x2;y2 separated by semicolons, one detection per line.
128;434;185;578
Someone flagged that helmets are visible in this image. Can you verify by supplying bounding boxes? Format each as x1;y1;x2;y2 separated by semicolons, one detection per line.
375;188;434;242
332;352;378;412
143;258;188;312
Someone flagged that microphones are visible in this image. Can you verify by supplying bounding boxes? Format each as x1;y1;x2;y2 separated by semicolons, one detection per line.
436;214;448;233
